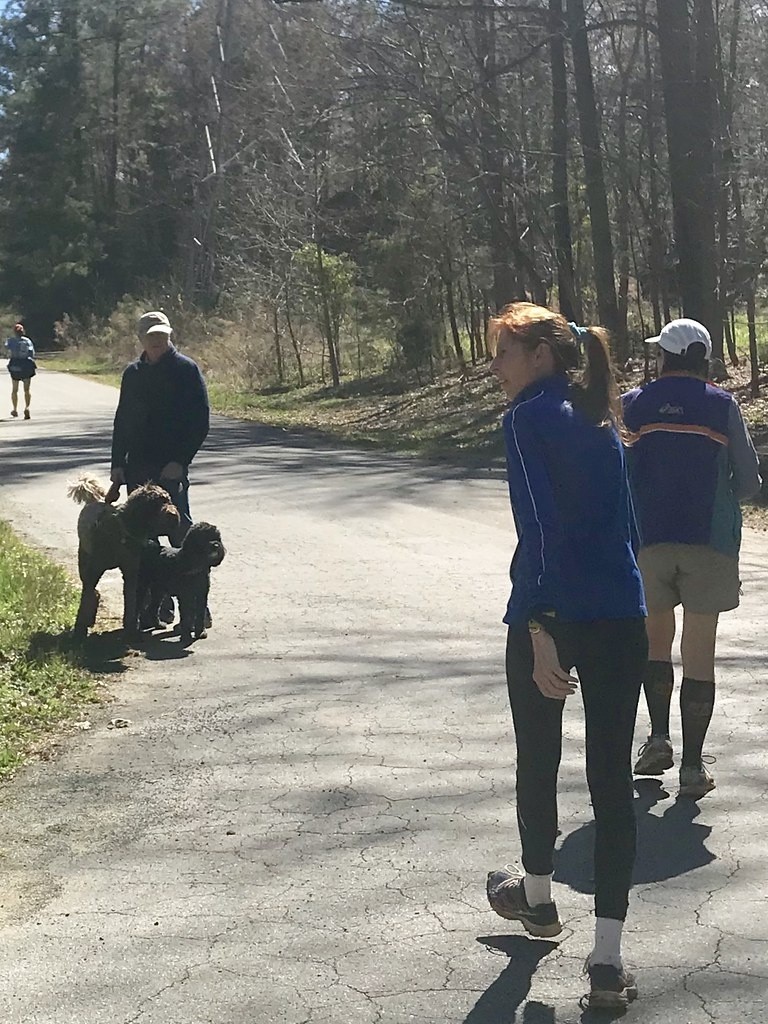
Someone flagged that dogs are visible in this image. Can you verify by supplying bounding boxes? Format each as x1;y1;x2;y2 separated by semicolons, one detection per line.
65;472;227;638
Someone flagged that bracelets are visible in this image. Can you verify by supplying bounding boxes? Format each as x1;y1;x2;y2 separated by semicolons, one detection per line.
526;608;560;639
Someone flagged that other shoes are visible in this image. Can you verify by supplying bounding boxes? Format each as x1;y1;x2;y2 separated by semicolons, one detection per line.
158;612;174;623
24;410;30;419
11;411;18;416
173;615;212;634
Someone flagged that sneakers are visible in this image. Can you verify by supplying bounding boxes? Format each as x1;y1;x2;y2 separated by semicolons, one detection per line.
679;755;717;793
583;952;637;1007
633;734;674;776
486;864;563;938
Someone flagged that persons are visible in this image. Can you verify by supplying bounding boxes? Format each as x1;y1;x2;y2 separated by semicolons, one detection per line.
612;318;762;795
5;323;37;419
109;311;213;634
487;301;672;1009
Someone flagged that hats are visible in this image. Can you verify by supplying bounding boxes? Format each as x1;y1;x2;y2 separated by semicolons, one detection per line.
138;311;170;336
644;319;712;360
